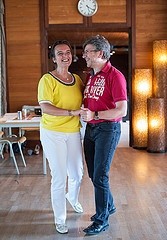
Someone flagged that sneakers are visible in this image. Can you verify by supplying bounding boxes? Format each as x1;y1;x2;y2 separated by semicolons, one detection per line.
56;225;68;234
67;195;83;212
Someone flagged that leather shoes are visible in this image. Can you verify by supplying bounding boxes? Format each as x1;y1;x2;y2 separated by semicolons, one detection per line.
91;206;116;221
83;221;109;234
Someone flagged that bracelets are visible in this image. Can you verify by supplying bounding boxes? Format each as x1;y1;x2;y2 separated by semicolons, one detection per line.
68;110;72;116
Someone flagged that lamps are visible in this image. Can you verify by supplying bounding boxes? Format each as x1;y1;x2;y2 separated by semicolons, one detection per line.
134;38;167;154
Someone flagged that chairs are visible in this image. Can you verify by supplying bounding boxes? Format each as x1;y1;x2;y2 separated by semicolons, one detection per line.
0;130;27;175
19;105;42;154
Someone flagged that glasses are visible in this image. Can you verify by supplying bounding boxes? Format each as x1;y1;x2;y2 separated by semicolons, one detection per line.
81;50;100;54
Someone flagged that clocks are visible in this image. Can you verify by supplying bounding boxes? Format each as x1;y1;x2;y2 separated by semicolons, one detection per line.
78;0;98;17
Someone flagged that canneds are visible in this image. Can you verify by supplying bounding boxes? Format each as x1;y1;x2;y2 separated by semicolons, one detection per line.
18;111;23;120
22;108;27;117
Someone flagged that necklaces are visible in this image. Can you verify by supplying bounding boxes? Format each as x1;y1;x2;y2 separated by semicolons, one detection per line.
58;71;69;80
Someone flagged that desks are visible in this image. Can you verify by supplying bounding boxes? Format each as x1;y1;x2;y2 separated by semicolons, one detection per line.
0;113;47;175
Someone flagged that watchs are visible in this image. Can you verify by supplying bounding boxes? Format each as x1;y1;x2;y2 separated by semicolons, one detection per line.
94;111;100;121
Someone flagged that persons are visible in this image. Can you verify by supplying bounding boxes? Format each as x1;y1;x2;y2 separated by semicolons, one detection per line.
81;35;128;234
37;40;85;234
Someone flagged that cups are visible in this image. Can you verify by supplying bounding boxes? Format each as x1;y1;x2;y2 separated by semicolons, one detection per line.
22;109;25;119
24;109;30;119
6;114;13;122
18;111;22;120
